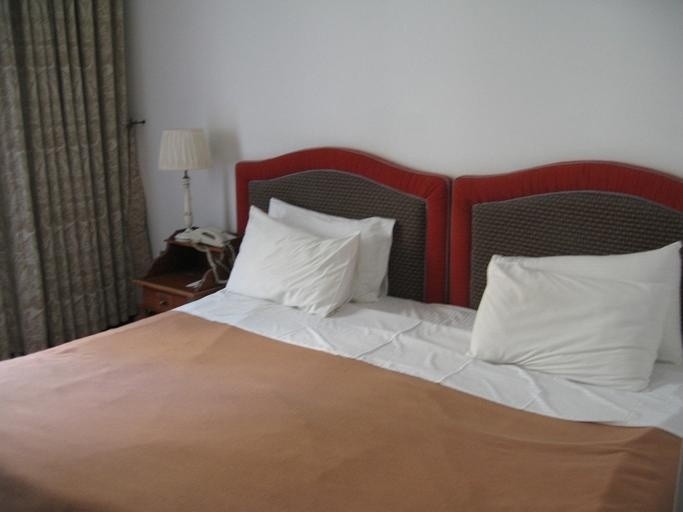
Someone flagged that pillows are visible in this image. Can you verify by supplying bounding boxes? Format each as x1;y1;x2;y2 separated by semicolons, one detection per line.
467;241;683;392
225;197;397;318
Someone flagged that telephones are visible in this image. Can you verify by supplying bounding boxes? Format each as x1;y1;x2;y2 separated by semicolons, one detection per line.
192;227;230;248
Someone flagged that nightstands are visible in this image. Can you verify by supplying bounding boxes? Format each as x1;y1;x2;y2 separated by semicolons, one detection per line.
129;227;242;322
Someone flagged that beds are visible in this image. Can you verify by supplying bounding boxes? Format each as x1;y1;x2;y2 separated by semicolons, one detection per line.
0;148;683;512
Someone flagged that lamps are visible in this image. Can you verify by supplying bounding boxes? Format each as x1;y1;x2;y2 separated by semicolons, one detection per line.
158;128;212;242
